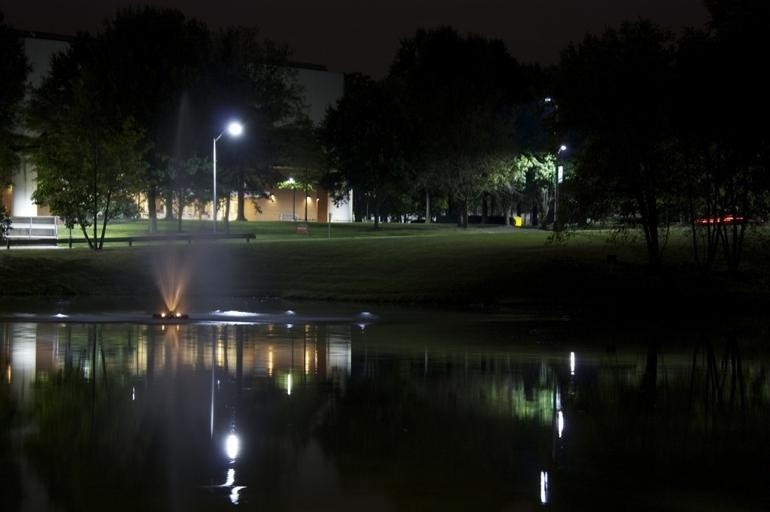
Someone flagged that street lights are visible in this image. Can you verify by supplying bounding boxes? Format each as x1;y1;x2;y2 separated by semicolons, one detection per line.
552;144;569;224
211;119;242;236
548;341;569;445
282;324;296;397
202;320;245;463
289;175;298;222
